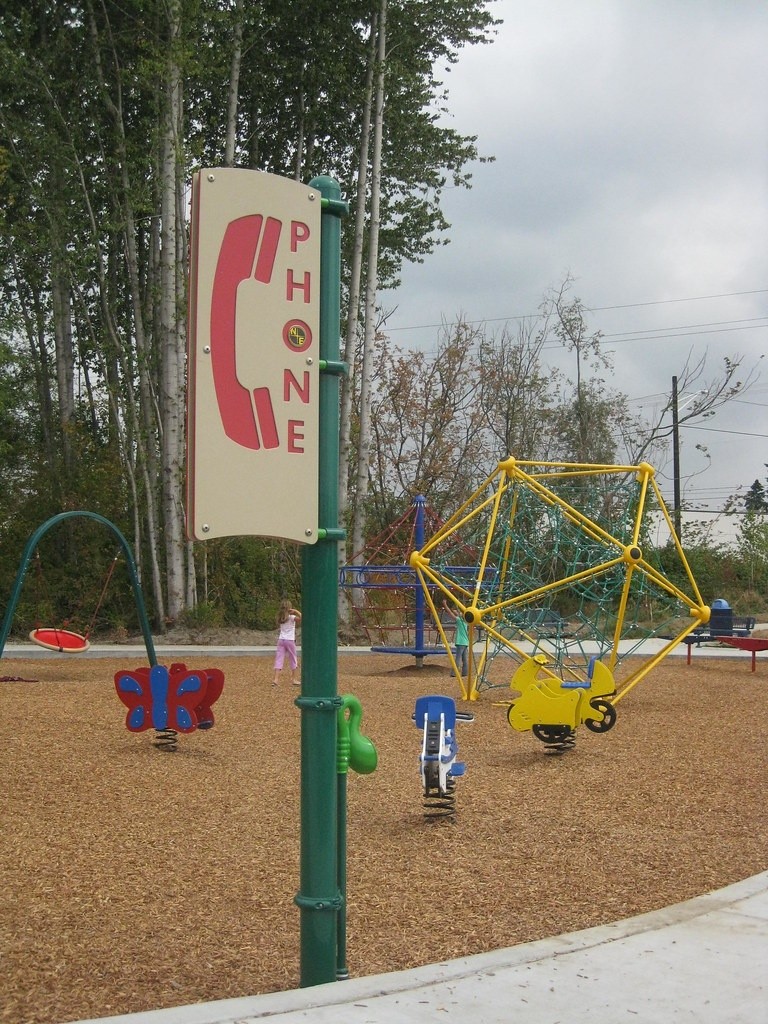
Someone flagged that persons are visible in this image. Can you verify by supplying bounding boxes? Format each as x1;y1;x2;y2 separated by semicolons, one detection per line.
271;600;302;686
442;599;470;677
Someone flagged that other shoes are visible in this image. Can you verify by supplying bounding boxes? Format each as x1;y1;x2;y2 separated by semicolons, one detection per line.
292;680;301;685
272;680;279;686
